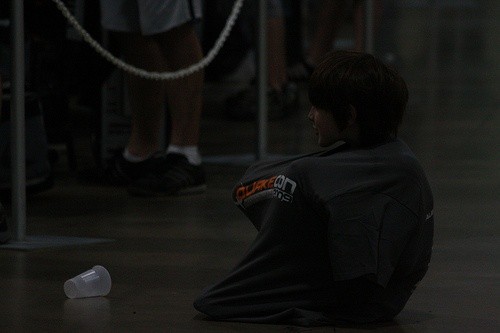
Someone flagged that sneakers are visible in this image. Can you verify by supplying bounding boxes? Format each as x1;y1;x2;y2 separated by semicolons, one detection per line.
77;146;165;189
127;151;208;199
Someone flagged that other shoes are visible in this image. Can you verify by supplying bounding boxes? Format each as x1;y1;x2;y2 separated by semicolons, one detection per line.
224;81;300;122
286;58;314;81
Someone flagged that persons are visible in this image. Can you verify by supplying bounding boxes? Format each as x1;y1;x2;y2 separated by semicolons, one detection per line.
194;46;434;328
87;0;209;196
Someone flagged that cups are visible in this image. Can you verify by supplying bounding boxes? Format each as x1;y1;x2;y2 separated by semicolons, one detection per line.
64;264;111;299
